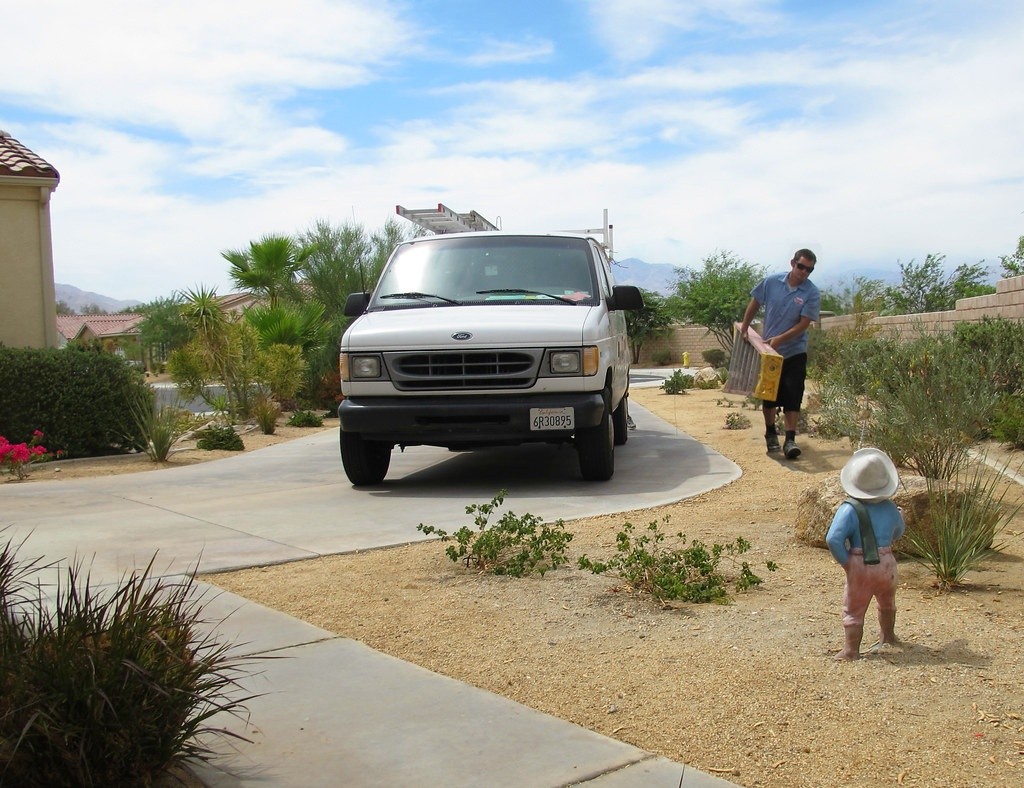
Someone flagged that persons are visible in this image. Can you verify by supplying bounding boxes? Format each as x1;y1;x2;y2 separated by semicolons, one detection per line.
827;447;906;661
740;249;820;459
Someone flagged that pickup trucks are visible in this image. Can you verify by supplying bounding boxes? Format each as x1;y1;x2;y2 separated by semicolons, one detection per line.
338;230;645;489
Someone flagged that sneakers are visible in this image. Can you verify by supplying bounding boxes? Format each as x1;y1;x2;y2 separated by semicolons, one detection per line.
783;441;801;459
763;433;781;452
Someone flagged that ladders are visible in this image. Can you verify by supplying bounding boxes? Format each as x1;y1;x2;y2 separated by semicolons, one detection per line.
396;204;498;233
717;322;783;401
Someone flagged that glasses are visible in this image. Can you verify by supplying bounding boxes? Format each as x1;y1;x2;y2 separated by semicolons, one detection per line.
794;260;814;273
